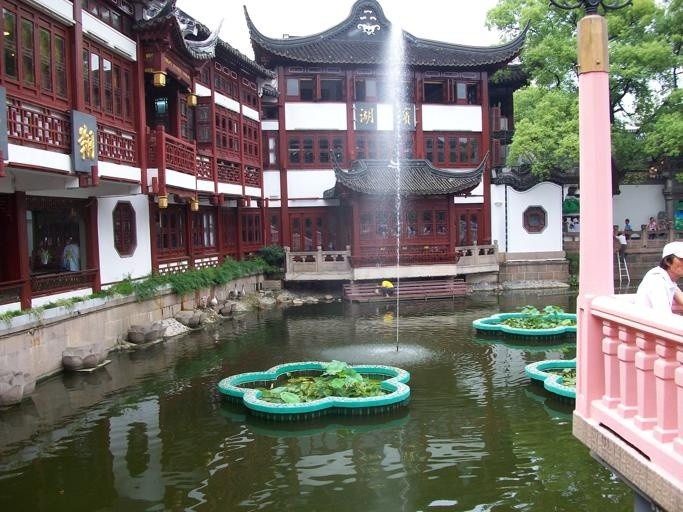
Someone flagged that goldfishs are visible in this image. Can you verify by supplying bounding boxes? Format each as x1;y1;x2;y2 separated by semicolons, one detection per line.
210;295;217;308
229;283;239;302
239;284;247;300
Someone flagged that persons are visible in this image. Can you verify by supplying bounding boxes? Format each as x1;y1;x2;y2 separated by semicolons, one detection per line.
633;240;683;319
58;235;80;274
613;232;627;262
624;219;632;240
381;302;395;322
376;278;394;298
561;217;579;242
645;217;658;239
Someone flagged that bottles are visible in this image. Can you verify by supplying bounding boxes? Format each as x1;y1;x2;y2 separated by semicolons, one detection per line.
662;241;682;258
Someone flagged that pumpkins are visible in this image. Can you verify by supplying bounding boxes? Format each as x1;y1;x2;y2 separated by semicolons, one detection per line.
617;250;630;284
